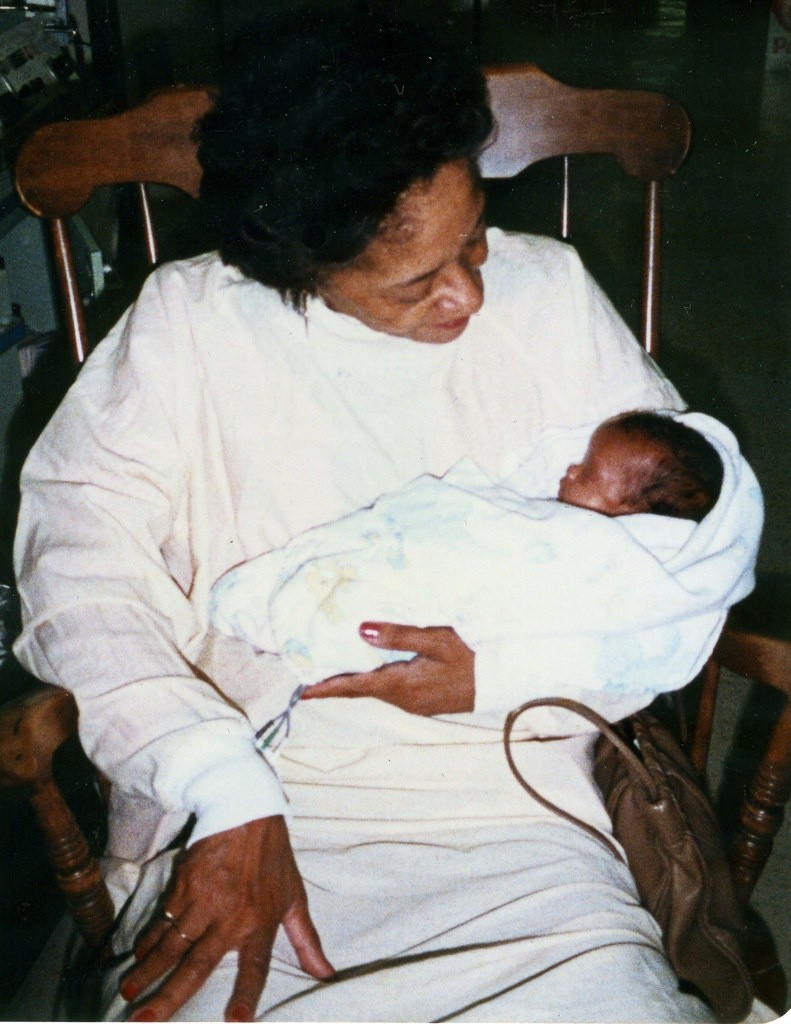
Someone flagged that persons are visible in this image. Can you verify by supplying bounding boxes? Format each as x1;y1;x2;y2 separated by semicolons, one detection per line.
11;20;714;1024
209;407;764;672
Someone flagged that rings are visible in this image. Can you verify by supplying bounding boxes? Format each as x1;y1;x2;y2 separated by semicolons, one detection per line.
161;910;176;925
173;922;196;944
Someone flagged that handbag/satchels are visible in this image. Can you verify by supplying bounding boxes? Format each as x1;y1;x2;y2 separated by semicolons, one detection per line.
502;700;788;1024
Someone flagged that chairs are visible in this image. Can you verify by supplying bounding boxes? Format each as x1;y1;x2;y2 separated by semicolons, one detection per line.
0;61;791;1024
